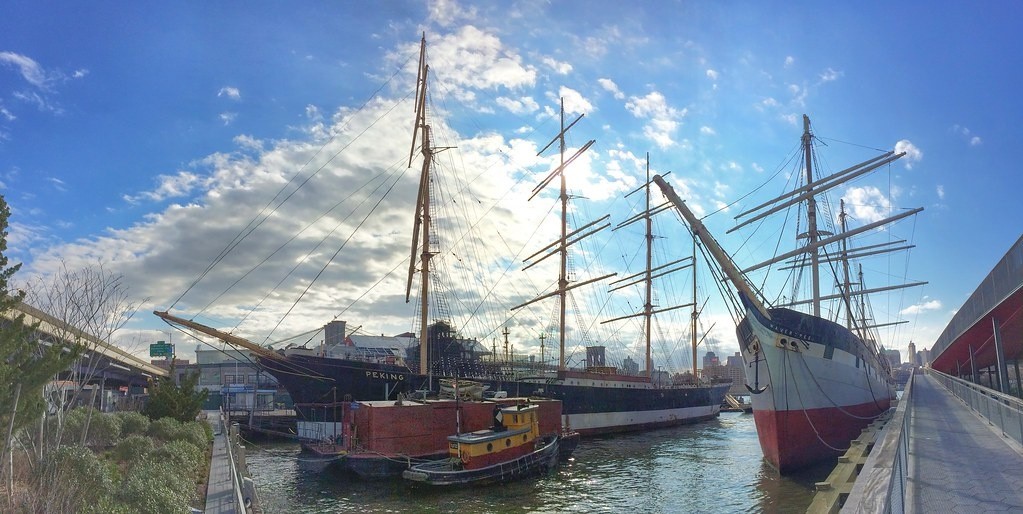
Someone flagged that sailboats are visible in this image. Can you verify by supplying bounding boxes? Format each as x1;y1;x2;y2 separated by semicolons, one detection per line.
152;29;753;443
652;113;931;481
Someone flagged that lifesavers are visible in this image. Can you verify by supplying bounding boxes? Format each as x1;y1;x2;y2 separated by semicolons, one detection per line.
461;449;470;463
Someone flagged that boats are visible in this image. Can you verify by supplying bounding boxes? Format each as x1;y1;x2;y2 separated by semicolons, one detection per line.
290;377;580;480
401;366;559;491
